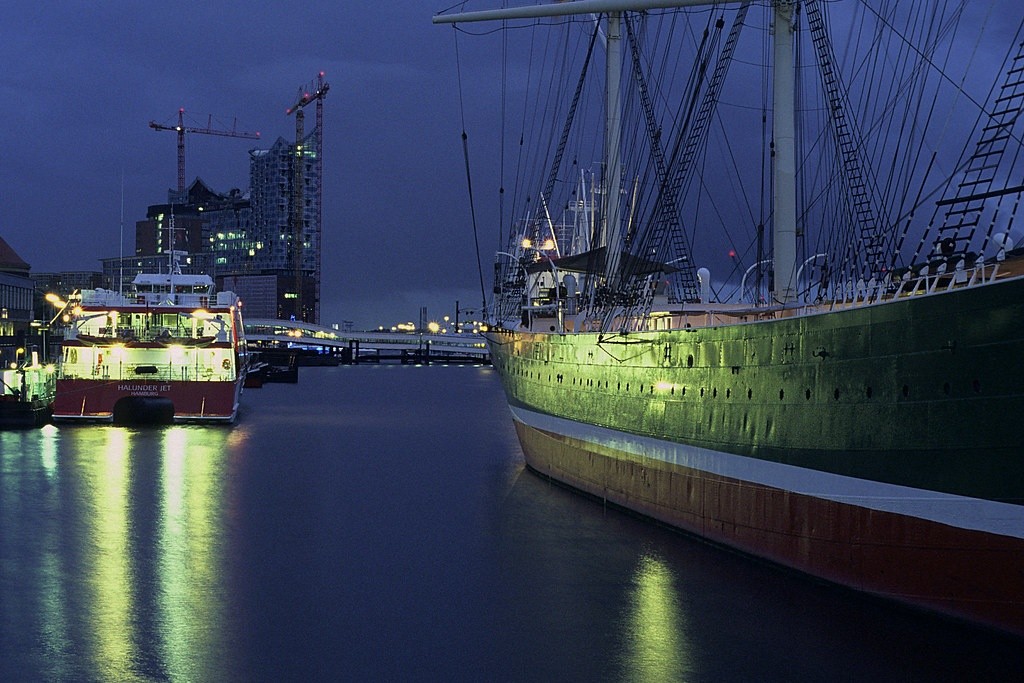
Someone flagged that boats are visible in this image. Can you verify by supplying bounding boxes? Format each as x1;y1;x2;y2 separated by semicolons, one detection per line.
49;203;270;426
0;352;59;428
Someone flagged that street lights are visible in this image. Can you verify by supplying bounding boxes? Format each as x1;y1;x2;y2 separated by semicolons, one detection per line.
15;348;25;365
42;294;60;364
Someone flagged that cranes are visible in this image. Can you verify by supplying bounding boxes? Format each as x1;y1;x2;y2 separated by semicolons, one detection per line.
150;107;261;203
285;72;332;326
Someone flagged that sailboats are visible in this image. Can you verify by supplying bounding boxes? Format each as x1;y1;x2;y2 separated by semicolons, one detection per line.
424;0;1024;634
